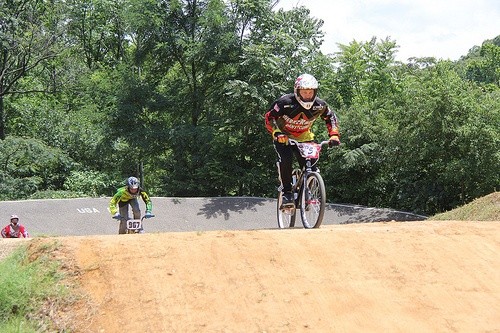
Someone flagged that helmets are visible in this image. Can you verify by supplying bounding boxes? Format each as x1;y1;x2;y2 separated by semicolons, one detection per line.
293;74;319;110
10;215;19;228
126;177;141;198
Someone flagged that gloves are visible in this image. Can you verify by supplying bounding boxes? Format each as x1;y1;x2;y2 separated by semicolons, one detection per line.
328;136;340;148
145;212;151;218
114;213;121;219
277;133;288;146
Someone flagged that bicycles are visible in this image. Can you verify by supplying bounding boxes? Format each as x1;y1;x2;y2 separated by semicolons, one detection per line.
273;137;331;229
113;214;155;234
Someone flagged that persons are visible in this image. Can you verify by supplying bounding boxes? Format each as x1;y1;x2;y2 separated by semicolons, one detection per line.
0;215;29;238
263;74;340;211
109;177;152;234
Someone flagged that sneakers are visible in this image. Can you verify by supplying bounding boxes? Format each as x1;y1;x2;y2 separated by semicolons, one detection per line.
282;190;295;212
